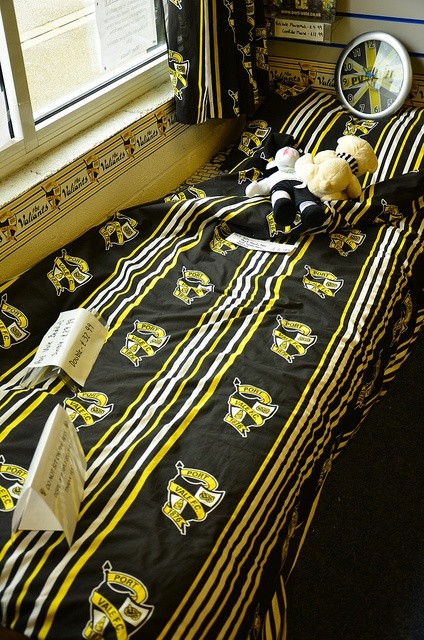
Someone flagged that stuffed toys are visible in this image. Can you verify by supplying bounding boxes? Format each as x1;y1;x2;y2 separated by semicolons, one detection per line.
293;134;379;203
244;145;325;228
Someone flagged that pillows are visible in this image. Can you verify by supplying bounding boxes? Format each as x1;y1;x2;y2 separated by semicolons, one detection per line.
277;84;424;191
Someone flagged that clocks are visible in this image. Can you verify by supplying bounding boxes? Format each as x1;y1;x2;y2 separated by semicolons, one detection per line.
334;31;413;120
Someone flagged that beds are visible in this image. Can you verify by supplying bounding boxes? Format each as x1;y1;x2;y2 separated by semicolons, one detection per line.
0;87;422;638
